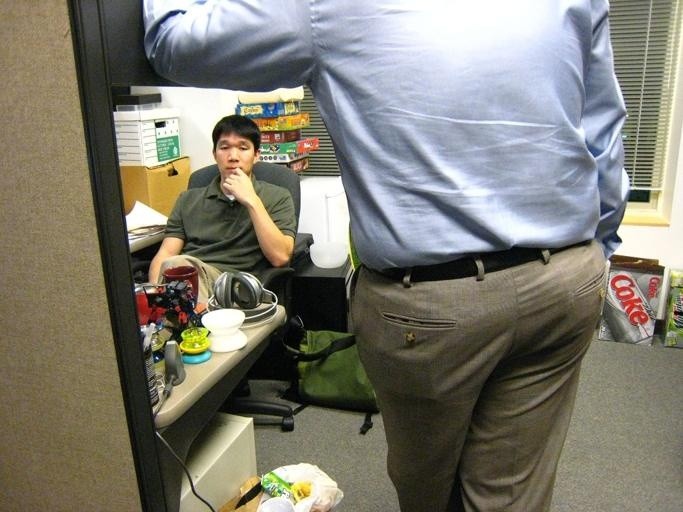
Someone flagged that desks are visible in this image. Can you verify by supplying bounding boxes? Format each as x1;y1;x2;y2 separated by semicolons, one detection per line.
122;212;288;511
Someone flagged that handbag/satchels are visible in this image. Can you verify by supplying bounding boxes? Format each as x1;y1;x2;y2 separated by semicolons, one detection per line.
297;330;380;413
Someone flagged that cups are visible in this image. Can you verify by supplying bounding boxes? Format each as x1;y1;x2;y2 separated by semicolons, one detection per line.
161;264;198;310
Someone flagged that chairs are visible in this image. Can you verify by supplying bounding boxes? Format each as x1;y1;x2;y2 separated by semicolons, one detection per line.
130;161;301;430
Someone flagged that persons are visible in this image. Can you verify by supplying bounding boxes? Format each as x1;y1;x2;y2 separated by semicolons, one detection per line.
146;115;298;307
143;0;629;512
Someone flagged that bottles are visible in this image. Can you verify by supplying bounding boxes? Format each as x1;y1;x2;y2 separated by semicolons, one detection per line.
146;324;165;391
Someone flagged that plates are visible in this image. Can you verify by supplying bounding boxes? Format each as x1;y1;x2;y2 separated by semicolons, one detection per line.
207;328;248;353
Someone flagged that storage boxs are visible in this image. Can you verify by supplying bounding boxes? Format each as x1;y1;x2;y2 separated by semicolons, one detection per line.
597;255;683;349
111;108;190;218
179;413;257;512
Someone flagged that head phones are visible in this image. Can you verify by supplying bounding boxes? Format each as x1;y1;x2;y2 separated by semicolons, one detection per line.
208;269;279;322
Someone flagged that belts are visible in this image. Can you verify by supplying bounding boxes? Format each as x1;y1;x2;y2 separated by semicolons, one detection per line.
376;242;590;284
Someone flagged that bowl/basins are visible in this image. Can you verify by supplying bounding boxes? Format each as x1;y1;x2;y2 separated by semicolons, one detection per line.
201;309;246;336
310;241;349;269
